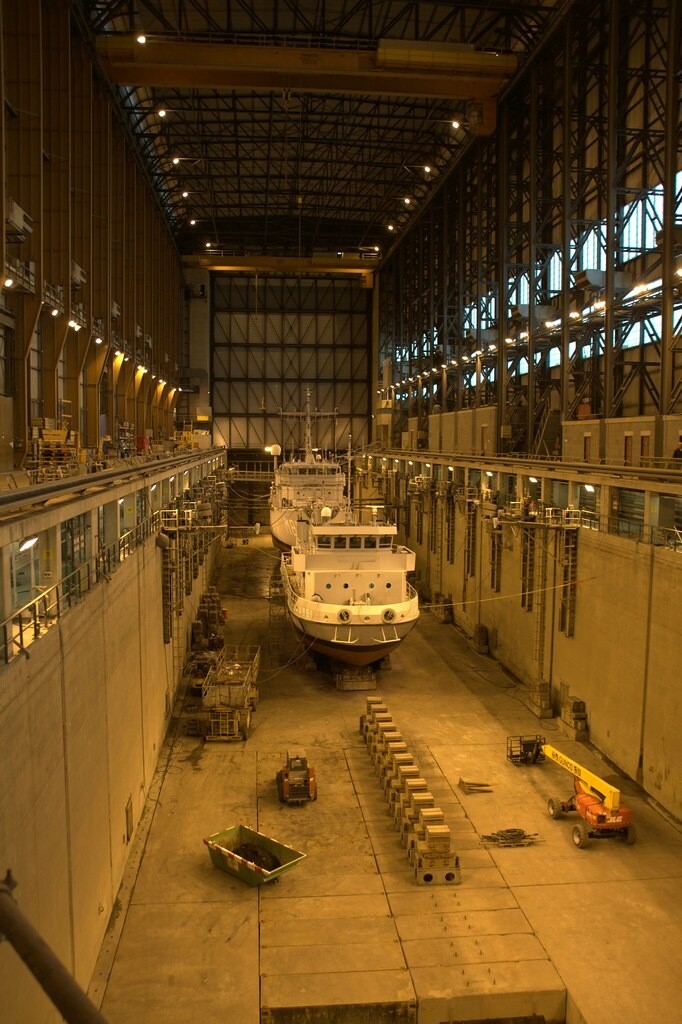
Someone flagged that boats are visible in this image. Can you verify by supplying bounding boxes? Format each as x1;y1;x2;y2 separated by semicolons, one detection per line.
267;390;354;557
279;432;421;666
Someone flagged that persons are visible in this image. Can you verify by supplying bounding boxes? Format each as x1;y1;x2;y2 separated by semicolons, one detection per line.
673;441;682;458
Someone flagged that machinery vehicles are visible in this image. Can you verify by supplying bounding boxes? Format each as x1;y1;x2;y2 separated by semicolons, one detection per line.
506;733;637;846
